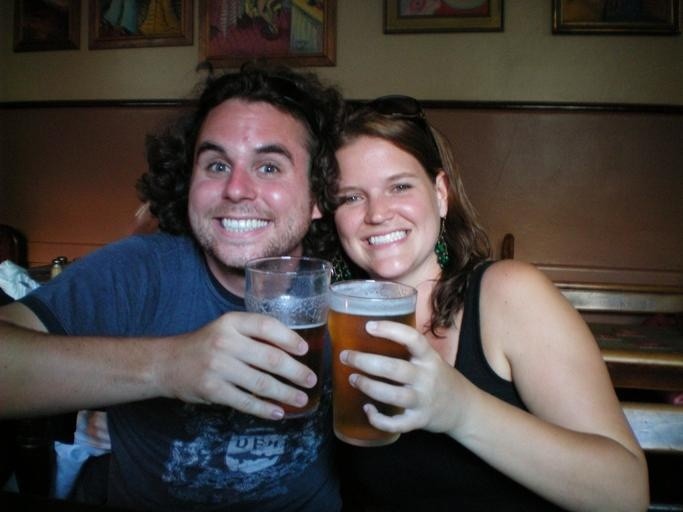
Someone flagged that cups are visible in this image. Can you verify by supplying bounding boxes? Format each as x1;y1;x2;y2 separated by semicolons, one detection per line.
242;256;418;449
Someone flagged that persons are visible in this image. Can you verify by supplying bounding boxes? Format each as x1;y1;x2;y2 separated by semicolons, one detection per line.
0;66;351;510
309;92;653;511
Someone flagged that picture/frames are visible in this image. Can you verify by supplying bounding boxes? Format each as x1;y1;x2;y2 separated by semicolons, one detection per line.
551;1;680;36
383;0;505;33
198;0;336;69
88;0;194;49
13;0;80;52
